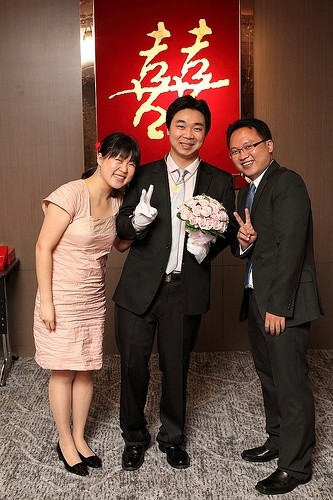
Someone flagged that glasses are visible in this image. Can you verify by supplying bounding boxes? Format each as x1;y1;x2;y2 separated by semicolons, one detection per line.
227;140;267;158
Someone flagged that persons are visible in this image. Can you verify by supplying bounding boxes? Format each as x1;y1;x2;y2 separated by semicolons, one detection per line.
226;118;326;495
33;133;142;476
113;96;236;470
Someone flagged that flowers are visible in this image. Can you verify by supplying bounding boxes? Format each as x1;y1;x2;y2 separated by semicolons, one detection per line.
177;194;230;239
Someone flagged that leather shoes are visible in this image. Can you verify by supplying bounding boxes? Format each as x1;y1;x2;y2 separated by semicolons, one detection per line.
241;442;279;462
159;442;190;469
122;435;151;471
255;469;312;495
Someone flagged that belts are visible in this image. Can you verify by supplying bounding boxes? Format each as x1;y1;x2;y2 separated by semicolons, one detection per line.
162;273;181;282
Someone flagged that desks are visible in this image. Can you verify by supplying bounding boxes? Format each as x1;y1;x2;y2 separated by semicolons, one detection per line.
0;258;22;386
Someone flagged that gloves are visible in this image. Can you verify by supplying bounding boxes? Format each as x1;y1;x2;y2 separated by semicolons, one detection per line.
132;184;158;228
187;233;210;264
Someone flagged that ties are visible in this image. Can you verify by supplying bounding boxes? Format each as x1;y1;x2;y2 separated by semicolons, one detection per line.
243;183;256;287
164;168;188;274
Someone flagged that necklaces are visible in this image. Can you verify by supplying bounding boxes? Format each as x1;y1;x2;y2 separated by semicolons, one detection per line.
163;153;201;185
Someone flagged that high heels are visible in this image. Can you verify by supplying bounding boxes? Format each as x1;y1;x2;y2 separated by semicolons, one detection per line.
78;442;102;468
56;439;89;476
